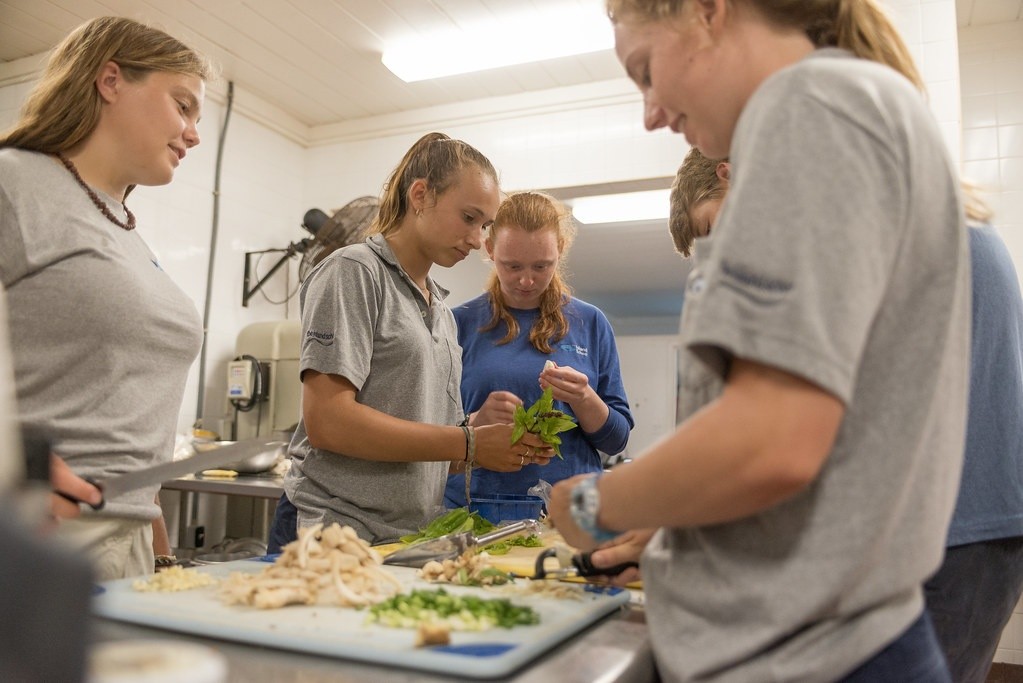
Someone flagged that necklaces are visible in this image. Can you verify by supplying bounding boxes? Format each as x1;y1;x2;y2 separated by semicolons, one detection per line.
56;152;136;230
401;265;427;291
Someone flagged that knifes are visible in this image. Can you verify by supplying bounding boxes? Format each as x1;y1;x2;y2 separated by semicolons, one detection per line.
53;431;285;515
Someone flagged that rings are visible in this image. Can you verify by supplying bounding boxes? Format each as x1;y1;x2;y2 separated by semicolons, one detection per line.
524;447;530;456
520;456;524;465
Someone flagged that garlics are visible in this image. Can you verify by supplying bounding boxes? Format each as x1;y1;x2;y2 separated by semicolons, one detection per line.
135;563;217;592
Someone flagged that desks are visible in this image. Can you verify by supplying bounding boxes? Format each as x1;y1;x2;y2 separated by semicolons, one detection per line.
153;475;293;550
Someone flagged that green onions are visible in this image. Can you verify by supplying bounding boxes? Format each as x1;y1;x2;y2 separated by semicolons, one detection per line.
371;586;542;628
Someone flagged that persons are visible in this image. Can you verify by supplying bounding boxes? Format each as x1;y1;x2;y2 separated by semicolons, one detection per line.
669;146;1023;683
0;17;207;584
547;1;975;682
266;132;498;555
441;193;635;523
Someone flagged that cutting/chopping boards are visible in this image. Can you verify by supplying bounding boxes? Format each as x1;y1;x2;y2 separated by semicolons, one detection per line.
369;519;645;591
83;545;633;679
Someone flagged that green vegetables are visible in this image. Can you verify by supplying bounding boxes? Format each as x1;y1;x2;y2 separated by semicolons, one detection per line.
511;387;577;460
401;510;543;553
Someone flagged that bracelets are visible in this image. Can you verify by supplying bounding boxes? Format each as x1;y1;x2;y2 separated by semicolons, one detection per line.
461;426;473;503
155;554;177;565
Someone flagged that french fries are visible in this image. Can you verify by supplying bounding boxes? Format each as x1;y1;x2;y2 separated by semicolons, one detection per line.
227;521;402;607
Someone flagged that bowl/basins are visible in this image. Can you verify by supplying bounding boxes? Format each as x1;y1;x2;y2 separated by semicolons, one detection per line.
192;439;288;473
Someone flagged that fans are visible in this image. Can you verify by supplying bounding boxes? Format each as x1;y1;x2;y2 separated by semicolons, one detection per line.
244;194;384;313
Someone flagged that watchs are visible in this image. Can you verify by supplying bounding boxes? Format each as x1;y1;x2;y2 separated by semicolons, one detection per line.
460;414;470;427
568;474;602;530
